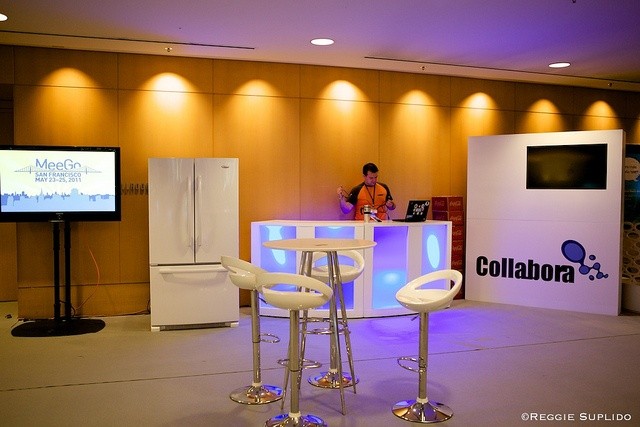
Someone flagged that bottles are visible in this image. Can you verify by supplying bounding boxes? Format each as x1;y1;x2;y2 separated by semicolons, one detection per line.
364;205;370;224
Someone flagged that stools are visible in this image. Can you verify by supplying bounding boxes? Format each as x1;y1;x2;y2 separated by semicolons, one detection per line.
306;250;365;389
221;253;283;404
260;273;334;427
391;270;462;423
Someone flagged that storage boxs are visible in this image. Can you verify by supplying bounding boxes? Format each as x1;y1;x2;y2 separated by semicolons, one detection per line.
432;212;465;226
432;194;463;212
452;241;464;301
452;227;465;241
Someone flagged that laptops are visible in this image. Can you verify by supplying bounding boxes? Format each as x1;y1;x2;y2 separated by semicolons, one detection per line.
392;200;430;221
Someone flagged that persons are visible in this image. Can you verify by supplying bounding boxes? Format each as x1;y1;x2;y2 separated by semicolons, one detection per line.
337;163;396;220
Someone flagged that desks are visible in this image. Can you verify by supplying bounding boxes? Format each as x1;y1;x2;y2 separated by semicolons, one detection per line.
264;239;376;414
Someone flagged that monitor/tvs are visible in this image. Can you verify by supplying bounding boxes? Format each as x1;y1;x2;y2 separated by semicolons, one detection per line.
0;143;122;223
